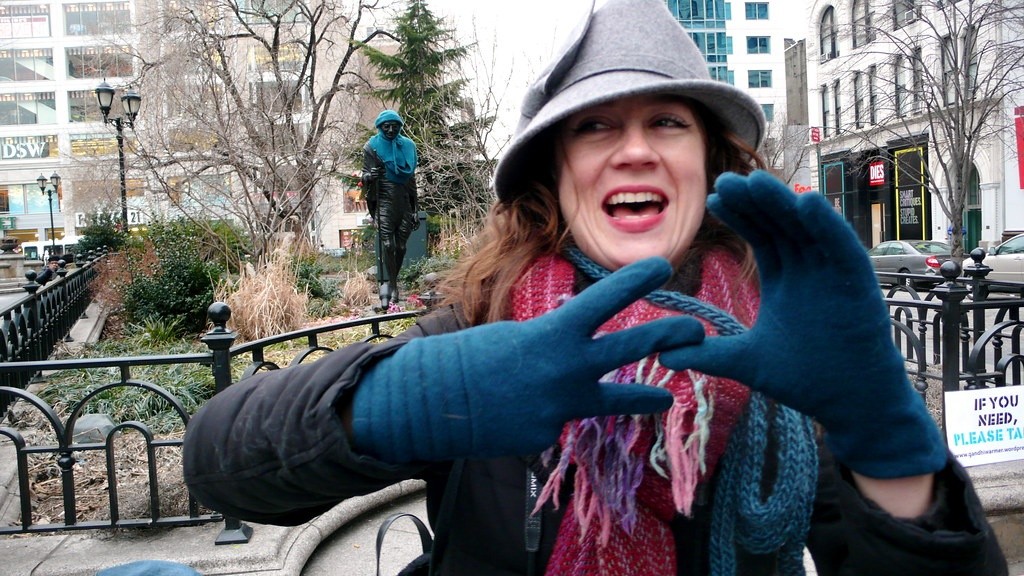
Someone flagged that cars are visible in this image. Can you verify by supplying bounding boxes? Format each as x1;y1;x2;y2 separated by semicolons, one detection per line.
961;233;1024;300
865;240;951;292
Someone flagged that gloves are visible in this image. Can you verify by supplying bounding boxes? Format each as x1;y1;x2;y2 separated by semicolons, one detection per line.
350;256;705;467
657;168;950;480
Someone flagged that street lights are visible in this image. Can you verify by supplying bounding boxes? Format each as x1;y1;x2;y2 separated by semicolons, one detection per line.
94;77;142;231
35;170;61;255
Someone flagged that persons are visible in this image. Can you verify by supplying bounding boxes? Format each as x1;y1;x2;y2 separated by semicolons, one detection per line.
185;0;1011;576
37;255;60;284
360;110;419;301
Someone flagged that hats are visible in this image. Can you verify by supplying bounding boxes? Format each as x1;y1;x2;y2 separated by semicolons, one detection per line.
493;0;766;202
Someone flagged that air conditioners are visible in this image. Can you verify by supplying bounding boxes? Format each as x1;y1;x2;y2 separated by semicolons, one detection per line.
905;7;917;24
937;0;950;10
821;52;831;63
